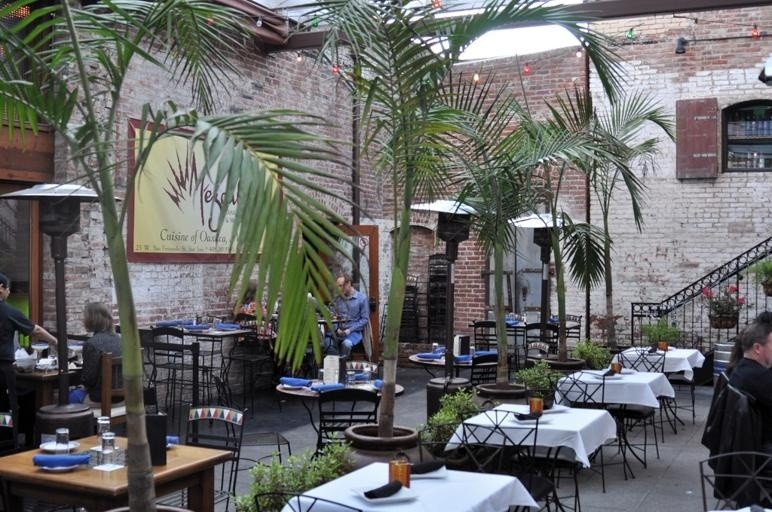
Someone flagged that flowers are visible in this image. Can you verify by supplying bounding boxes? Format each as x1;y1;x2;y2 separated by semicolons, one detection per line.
700;285;746;315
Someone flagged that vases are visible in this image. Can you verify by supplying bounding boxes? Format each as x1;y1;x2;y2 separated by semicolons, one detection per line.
707;312;739;330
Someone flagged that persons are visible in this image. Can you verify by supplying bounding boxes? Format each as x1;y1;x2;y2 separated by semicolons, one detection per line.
724;328;745;380
324;276;370;360
730;324;772;509
233;279;276;340
0;272;74;458
66;300;127;408
263;282;278;313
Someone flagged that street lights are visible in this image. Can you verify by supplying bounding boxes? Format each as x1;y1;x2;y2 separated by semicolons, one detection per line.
411;201;505;432
411;201;479;403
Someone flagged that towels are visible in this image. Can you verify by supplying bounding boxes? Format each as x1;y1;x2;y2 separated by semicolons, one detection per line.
648;345;657;352
164;434;179;445
455;351;470;363
311;382;345;391
154;319;179;328
375;379;384;387
432;345;448;352
505;319;520;326
33;453;91;465
185;323;212;328
475;350;497;356
215;323;241;328
411;457;446;474
366;480;402;498
179;318;194;325
515;410;541;420
603;369;614;376
279;376;312;387
416;352;443;359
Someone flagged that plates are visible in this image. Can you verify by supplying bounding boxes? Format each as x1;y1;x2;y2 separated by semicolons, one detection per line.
542;405;567;415
359;482;413;504
307;389;326;395
596;372;621;380
282;384;303;390
418;355;434;362
39;466;80;475
515;418;552;425
39;440;80;451
413;464;450;478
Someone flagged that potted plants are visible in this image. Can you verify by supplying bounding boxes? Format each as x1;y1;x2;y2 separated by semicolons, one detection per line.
513;361;566;410
746;258;772;299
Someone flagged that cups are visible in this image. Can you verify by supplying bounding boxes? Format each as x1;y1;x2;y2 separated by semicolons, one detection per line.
346;371;356;386
88;444;120;465
318;368;326;384
432;343;441;351
57;427;72;454
97;417;113;441
102;431;113;450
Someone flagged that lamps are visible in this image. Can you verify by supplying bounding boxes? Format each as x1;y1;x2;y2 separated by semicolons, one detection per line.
408;201;479;377
508;212;568;354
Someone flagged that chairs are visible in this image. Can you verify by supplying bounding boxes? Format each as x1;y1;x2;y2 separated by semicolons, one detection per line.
526;322;561;361
93;346;147;429
615;344;685;435
566;314;583;341
343;359;381;426
154;404;246;512
468;353;499;389
461;408;539;512
524;374;592;476
472;319;498;350
417;421;507;475
166;310;233;409
700;453;772;510
148;324;186;438
223;312;283;420
308;387;382;461
507;324;525;372
606;405;659;470
254;491;362;512
546;370;645;494
